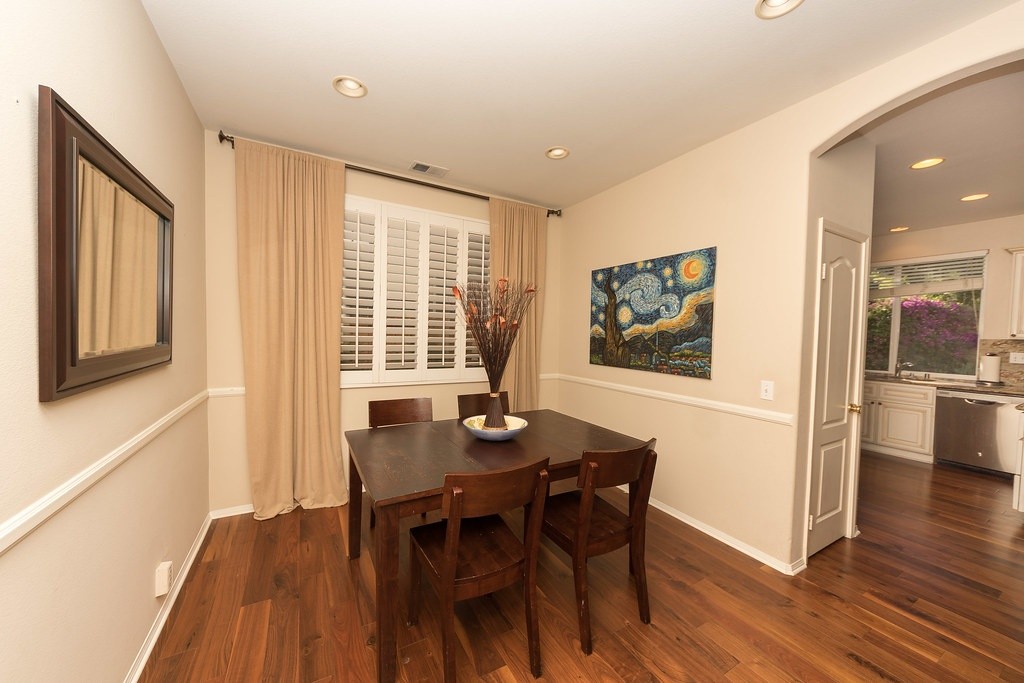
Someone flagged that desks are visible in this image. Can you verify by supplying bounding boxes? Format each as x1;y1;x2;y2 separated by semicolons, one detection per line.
343;408;649;683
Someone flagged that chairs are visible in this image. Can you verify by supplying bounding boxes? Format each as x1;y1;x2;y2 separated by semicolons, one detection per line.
368;398;433;547
406;457;549;683
523;437;657;655
457;391;510;419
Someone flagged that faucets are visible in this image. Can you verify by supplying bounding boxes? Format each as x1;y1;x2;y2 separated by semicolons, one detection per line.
895;358;914;377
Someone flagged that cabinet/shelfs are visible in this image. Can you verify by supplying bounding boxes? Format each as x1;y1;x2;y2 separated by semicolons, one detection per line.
862;381;936;463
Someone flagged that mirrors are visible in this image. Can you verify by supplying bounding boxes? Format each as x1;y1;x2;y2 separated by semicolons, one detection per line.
38;84;176;403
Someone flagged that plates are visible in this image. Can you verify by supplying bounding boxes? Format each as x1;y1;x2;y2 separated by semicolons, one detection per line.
463;414;528;440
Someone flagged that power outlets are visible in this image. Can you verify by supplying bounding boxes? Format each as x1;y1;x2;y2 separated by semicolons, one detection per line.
760;380;774;401
154;561;174;598
